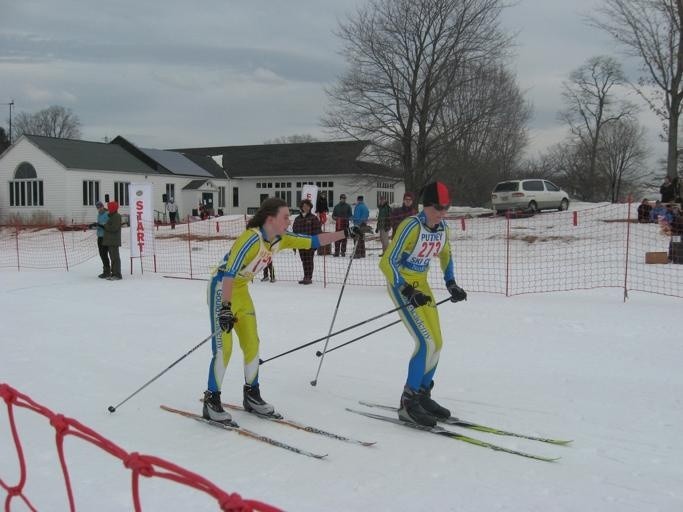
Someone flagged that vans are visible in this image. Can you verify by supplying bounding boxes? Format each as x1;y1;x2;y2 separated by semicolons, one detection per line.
490;178;569;215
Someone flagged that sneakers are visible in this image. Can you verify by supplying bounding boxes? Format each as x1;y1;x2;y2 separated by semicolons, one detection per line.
99;273;122;280
332;252;366;258
261;278;312;284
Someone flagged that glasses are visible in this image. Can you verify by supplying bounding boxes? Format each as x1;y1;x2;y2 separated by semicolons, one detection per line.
434;204;450;211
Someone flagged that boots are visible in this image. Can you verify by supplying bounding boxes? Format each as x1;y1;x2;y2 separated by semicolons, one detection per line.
398;386;437;427
203;390;231;422
420;381;451;417
243;383;275;415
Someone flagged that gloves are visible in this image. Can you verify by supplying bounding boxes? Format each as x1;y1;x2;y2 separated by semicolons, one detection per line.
375;228;378;233
446;281;466;302
219;303;235;332
88;222;102;230
343;226;366;241
402;284;427;308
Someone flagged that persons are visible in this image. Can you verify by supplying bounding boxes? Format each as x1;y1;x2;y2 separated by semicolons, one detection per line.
197;196;373;425
165;197;178;230
259;261;276;283
91;200;111;279
102;202;124;280
374;195;391;257
635;176;682;236
291;198;321;285
346;195;369;259
390;191;417;239
314;192;329;227
331;193;352;258
377;180;466;432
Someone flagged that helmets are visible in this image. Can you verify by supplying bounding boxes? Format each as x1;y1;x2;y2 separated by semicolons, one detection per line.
108;201;118;214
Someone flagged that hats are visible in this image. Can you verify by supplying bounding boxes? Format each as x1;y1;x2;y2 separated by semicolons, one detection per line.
95;202;103;207
422;181;453;206
403;192;415;200
